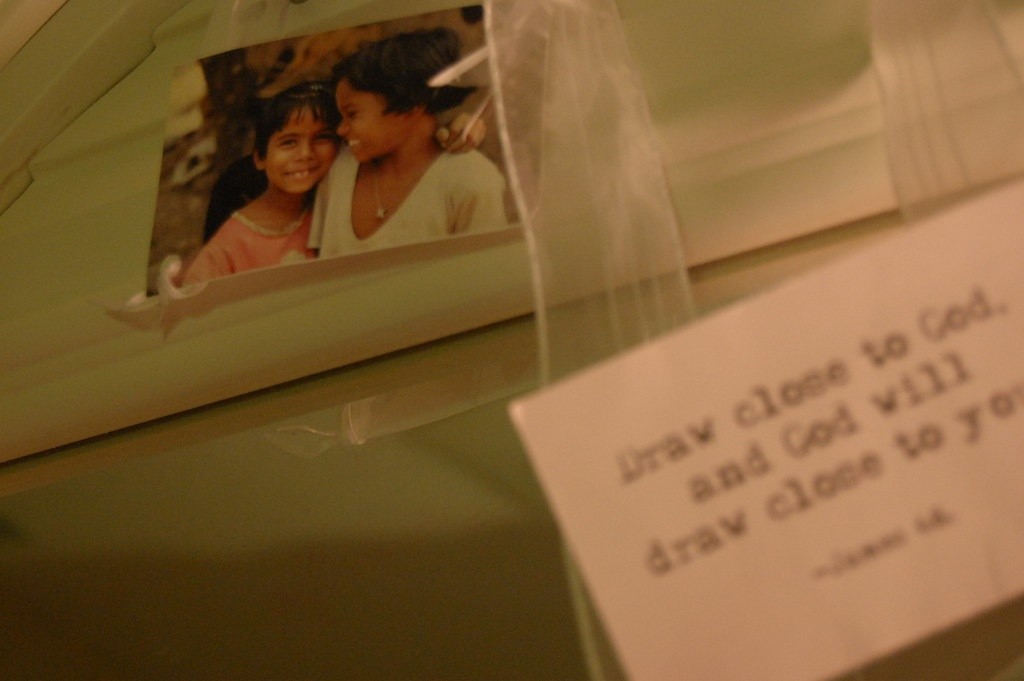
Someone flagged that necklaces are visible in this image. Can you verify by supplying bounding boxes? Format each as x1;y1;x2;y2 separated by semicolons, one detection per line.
374;173;389;218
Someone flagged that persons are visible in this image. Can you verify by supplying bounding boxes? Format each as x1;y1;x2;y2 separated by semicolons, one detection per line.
307;26;507;261
182;80;488;287
